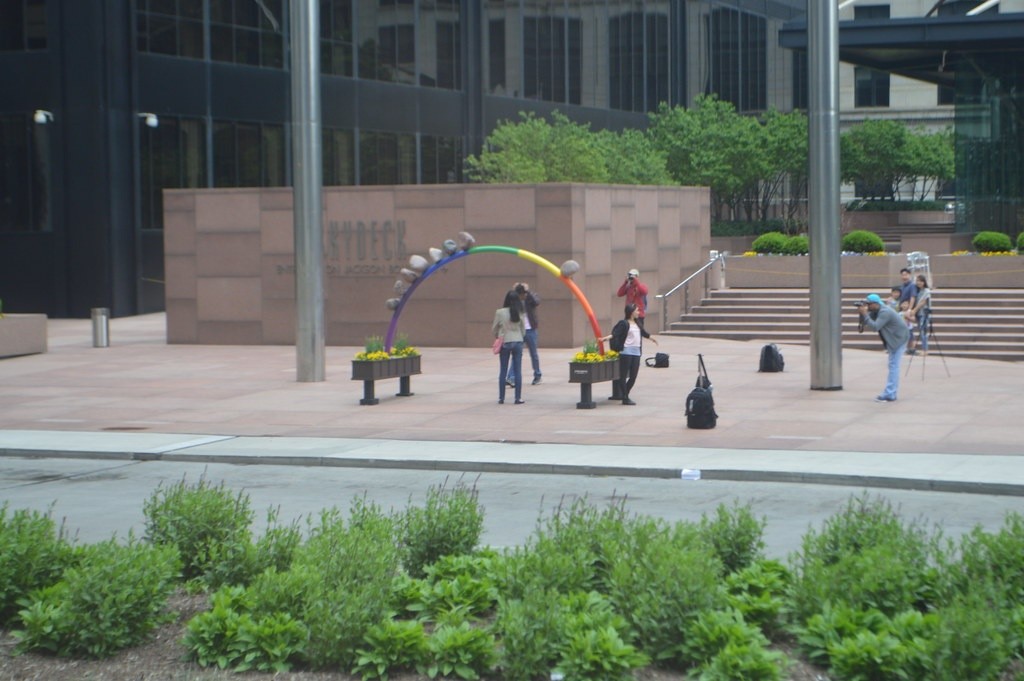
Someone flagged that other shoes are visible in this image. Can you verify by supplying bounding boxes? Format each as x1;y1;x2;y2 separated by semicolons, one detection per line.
532;377;541;385
506;377;515;387
874;393;897;403
499;399;503;404
622;396;636;405
515;399;525;404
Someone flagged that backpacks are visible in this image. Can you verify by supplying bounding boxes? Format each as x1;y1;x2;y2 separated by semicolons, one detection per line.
609;320;628;350
760;343;784;372
684;388;715;429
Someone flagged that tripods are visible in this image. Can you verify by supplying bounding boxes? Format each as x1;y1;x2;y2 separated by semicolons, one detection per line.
905;313;951;381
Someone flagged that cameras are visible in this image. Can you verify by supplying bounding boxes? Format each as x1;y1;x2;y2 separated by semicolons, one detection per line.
854;300;865;307
628;277;633;280
924;307;931;313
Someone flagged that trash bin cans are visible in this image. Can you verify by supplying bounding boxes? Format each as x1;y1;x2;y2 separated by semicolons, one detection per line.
90;307;110;348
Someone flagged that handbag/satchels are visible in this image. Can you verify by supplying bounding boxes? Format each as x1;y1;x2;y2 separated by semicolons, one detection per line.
493;335;504;354
645;353;669;367
695;353;711;388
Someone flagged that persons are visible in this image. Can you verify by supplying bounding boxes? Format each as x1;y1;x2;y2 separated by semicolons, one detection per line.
860;293;911;402
616;269;647;328
914;274;933;355
886;288;902;312
505;282;542;388
896;269;918;310
596;303;658;405
492;290;527;404
898;299;919;356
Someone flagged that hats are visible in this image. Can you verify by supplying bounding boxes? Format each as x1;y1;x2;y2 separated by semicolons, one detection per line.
867;294;883;305
627;269;639;277
